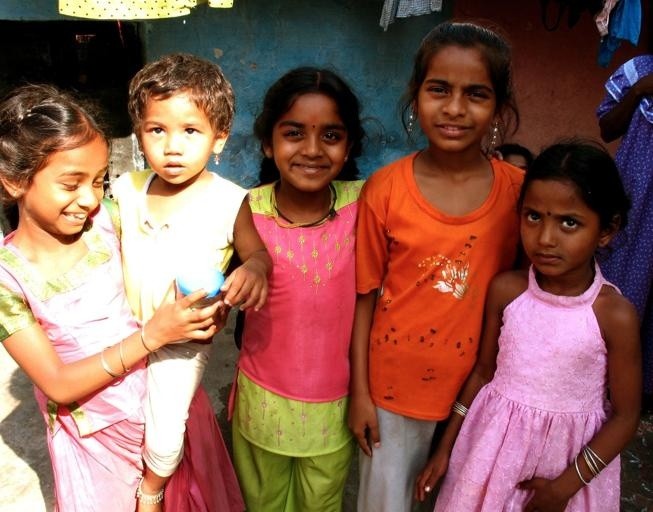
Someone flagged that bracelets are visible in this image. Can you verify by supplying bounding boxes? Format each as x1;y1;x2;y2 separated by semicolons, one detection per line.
452;400;468;417
141;320;160;353
102;342;131;377
574;446;608;486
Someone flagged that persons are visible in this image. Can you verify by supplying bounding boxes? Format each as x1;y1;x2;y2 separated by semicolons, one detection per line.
492;143;536;170
108;52;273;511
1;83;246;511
409;136;640;512
227;65;369;511
344;22;529;511
598;26;652;419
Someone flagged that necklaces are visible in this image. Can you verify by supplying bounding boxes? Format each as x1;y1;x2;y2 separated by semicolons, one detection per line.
275;181;338;228
